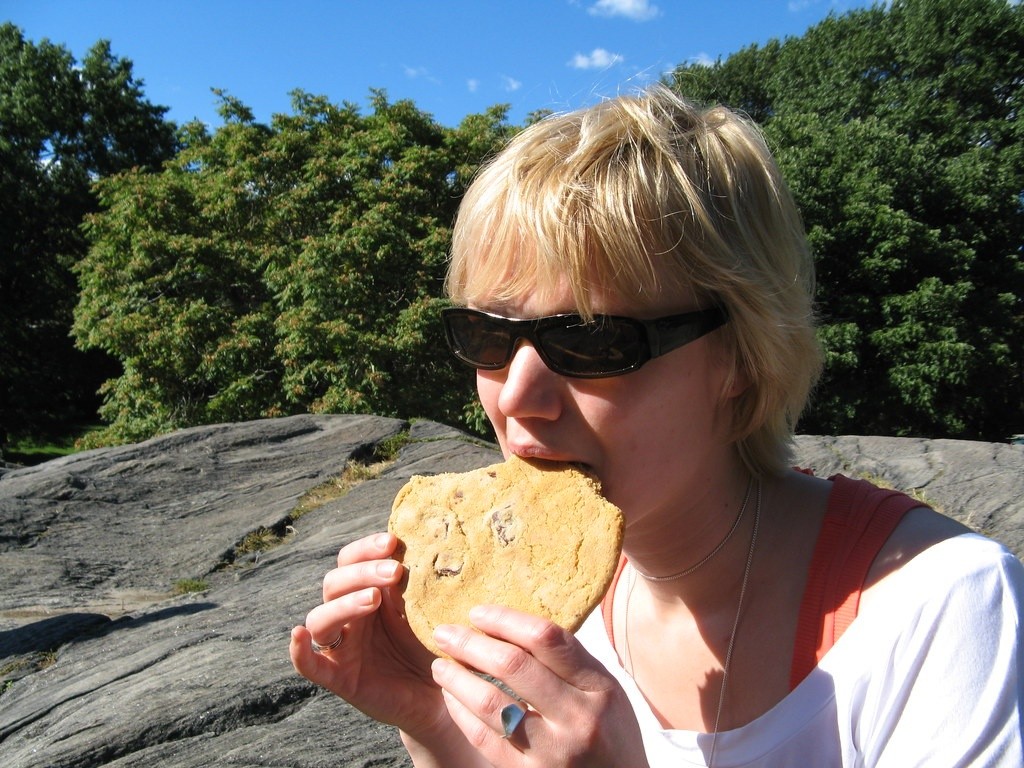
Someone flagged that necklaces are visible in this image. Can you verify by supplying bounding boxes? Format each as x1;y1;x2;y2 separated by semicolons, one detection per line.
623;477;762;768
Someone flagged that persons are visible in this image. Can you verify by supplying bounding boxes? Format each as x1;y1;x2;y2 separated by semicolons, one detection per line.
289;79;1024;768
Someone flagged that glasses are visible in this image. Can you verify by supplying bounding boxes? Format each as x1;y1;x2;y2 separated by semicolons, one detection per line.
440;292;732;379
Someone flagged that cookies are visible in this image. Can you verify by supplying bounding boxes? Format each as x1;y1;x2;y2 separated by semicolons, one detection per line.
383;458;625;669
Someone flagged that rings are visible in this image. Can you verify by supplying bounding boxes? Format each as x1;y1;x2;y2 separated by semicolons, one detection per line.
501;702;528;739
312;633;342;652
